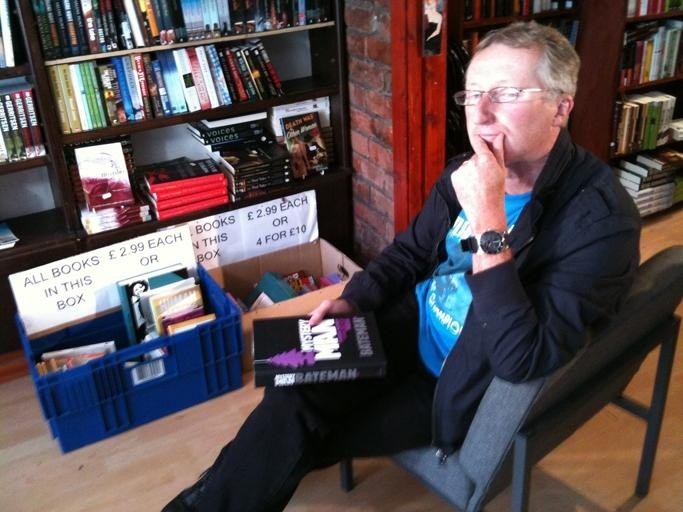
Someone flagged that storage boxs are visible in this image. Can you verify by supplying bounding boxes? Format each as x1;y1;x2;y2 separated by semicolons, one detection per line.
206;240;364;375
16;262;246;453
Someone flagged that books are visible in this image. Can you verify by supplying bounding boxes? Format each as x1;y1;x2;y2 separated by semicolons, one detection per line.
222;269;338;314
41;341;116;376
118;262;216;346
47;38;284;134
33;0;331;60
0;82;46;164
0;0;15;67
250;310;389;390
0;221;19;250
69;97;332;235
443;0;683;218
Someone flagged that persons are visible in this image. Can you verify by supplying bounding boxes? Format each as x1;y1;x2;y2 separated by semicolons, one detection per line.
159;19;647;510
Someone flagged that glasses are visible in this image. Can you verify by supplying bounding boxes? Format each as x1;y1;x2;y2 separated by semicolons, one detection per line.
452;85;559;108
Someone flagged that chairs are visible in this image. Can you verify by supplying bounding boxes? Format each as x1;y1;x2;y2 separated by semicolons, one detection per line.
337;247;683;512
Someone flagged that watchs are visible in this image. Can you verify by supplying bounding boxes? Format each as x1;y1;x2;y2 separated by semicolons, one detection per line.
456;226;512;256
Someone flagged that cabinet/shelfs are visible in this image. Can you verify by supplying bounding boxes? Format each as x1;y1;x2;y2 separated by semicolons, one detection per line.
0;1;683;347
